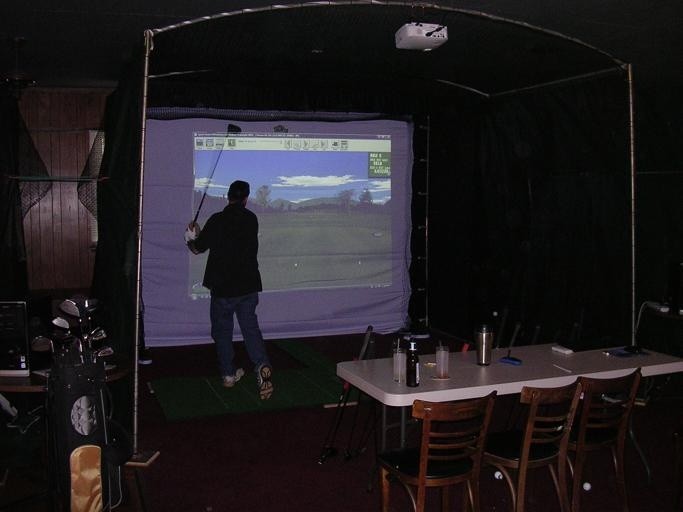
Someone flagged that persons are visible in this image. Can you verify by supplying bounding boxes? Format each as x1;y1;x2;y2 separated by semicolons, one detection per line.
181;178;274;404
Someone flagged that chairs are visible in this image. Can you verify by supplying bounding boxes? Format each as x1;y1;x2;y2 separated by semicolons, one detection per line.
371;369;645;512
44;374;134;511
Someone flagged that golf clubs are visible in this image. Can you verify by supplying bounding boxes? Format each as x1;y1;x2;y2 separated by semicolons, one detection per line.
320;326;378;467
33;293;114;368
192;125;242;227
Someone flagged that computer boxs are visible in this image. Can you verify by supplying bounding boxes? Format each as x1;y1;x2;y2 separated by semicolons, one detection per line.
1;297;35;378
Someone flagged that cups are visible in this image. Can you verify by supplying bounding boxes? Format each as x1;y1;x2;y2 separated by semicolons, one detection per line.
393;348;407;383
436;346;449;379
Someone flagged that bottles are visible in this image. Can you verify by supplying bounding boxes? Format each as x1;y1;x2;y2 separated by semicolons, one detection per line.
406;342;420;387
478;324;493;366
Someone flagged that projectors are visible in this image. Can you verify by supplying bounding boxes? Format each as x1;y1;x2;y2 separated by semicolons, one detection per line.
395;22;449;52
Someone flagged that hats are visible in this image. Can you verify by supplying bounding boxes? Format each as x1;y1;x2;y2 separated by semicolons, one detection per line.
228;180;249;200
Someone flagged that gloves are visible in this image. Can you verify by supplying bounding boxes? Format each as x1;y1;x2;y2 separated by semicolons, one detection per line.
184;221;201;245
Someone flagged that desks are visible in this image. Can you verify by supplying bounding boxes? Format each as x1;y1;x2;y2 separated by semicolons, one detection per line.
336;340;683;489
0;351;135;480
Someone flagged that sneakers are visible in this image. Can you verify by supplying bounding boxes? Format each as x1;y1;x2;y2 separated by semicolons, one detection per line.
258;364;273;399
224;367;245;387
